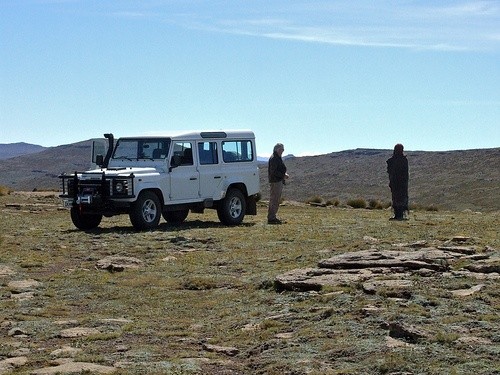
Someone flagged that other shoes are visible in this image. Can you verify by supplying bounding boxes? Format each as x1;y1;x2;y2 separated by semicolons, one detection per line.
389;217;402;221
268;218;287;224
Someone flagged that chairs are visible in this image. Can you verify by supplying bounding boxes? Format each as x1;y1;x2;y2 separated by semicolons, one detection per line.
153;149;163;157
180;148;193;165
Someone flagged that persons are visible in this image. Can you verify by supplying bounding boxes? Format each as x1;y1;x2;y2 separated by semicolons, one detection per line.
267;143;289;224
386;144;409;220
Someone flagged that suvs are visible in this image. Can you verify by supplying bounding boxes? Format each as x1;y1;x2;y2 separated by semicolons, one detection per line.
57;127;263;232
